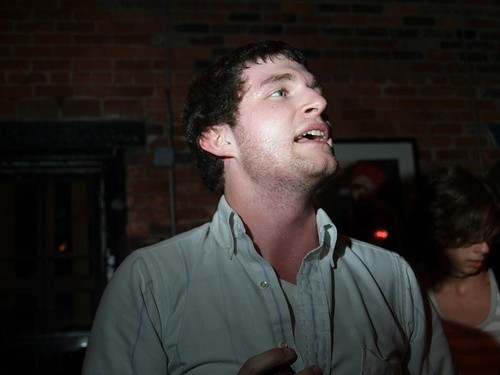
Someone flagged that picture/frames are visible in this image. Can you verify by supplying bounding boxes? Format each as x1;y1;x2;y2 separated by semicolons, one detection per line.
313;137;421;244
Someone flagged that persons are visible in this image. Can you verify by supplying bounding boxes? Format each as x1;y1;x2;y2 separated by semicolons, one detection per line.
411;165;500;375
81;38;455;375
